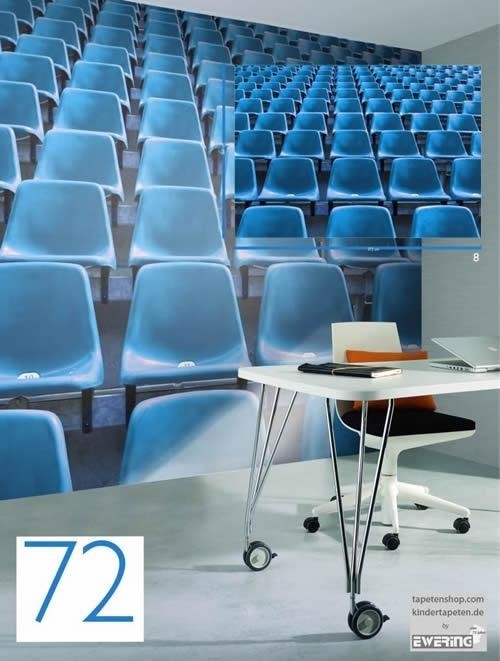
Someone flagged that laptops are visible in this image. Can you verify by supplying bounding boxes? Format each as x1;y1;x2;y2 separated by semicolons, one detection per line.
428;336;500;372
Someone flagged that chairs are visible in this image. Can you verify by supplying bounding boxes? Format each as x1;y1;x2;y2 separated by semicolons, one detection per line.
301;322;477;549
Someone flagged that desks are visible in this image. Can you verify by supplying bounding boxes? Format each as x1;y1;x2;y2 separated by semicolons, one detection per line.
237;357;500;639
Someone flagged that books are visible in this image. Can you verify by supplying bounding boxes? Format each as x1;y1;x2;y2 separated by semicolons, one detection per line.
298;359;404;379
